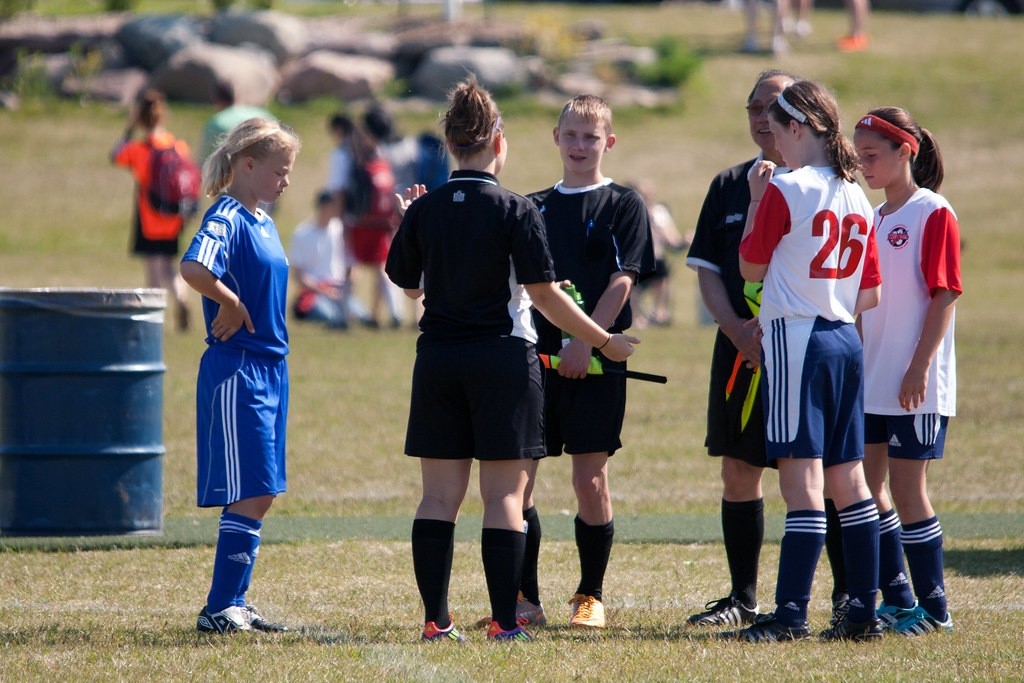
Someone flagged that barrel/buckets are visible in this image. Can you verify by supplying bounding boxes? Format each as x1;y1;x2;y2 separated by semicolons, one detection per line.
0;281;171;538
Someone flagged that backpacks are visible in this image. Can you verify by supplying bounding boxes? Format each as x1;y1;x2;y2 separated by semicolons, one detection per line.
347;159;395;223
412;134;450;200
147;144;200;217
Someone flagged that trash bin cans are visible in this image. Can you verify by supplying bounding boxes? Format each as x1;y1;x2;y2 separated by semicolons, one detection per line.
0;288;167;538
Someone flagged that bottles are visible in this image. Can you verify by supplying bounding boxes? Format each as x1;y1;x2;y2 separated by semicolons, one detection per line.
559;283;589;347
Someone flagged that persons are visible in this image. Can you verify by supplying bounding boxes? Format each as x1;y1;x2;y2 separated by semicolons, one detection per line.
683;69;855;637
835;0;872;54
854;108;963;635
776;0;813;37
384;75;642;643
180;116;301;633
741;0;790;53
736;80;883;643
109;82;690;332
472;94;656;627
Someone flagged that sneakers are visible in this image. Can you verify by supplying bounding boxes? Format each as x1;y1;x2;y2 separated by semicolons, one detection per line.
422;614;469;642
831;592;853;627
195;603;268;635
888;605;954;637
238;606;288;632
474;600;547;628
568;595;606;629
486;618;535;643
683;595;760;627
875;597;919;630
820;614;883;642
715;612;812;644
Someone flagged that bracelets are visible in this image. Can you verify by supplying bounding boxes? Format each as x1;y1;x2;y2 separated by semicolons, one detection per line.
750;200;760;202
598;333;611;351
